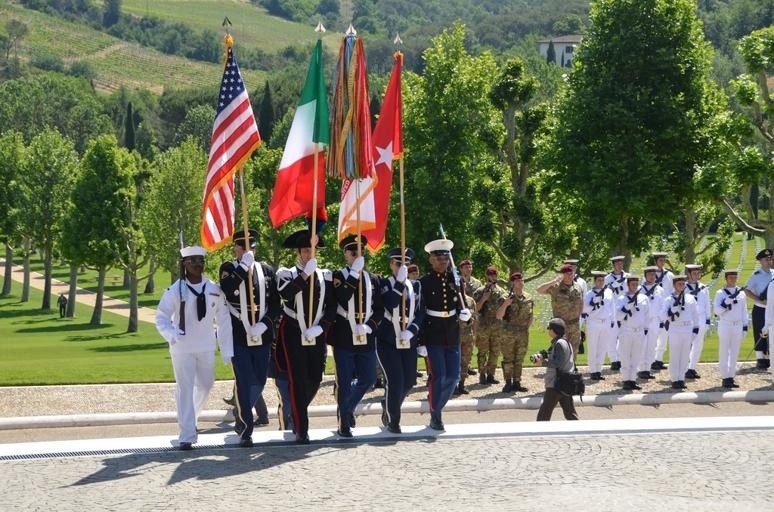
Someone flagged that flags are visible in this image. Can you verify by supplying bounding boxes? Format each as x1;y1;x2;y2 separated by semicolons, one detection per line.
200;35;404;253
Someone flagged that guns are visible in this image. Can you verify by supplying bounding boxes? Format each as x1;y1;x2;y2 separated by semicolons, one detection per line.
760;277;774;301
439;222;475;326
179;226;185;335
462;270;515;322
573;271;748;331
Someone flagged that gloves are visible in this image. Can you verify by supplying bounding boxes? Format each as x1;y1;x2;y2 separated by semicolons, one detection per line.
221;355;232;365
725;295;735;305
246;307;472;340
593;296;603;303
671;305;678;314
397;264;407;282
624;303;633;310
163;328;186;343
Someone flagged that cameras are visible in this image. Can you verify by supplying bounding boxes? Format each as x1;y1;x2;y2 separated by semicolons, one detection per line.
531;350;548;362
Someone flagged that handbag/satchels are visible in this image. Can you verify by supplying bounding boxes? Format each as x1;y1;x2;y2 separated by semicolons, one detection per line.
556;373;584;396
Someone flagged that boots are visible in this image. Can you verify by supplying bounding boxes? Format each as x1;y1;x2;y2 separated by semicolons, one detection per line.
458;367;527;394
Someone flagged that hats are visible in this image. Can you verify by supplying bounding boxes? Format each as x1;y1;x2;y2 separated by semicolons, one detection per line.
408;265;418;272
549;319;566;335
756;249;772;259
561;252;739;282
460;260;522;279
180;231;454;263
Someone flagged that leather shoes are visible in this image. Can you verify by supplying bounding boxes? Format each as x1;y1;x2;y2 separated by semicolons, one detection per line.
179;396;444;450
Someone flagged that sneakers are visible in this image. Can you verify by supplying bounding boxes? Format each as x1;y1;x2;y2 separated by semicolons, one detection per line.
590;359;770;390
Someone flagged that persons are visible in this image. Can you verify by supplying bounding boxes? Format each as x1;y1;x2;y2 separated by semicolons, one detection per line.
538;250;774;391
269;314;293;430
222;376;269;426
535;318;580;420
406;265;420;280
274;230;334;444
152;247;235;450
414;239;472;430
375;248;425;433
220;230;273;449
57;294;66;317
325;235;384;437
457;262;534;395
